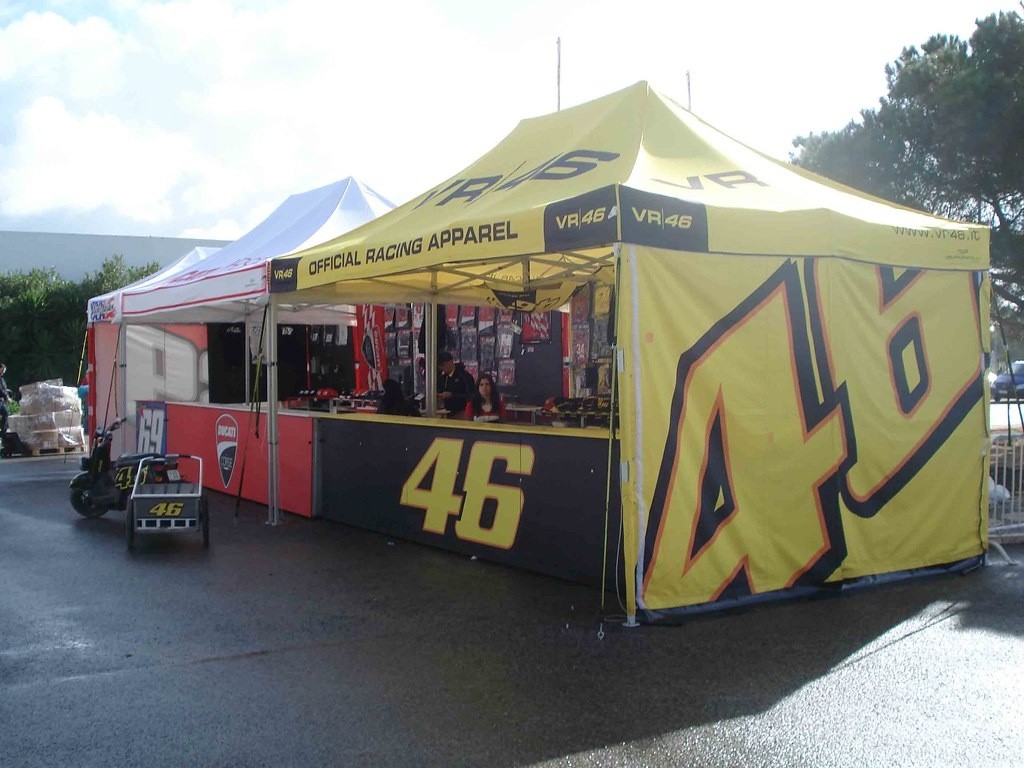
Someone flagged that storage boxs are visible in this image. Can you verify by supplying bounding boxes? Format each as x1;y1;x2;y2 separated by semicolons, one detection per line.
6;381;85;448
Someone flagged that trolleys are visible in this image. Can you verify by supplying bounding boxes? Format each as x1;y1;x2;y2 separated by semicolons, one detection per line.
125;451;210;554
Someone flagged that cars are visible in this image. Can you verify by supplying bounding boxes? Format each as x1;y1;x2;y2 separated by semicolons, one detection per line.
990;361;1024;403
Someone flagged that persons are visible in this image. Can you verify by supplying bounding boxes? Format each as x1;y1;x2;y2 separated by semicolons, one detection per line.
464;375;506;422
376;379;425;417
0;364;12;439
436;352;475;415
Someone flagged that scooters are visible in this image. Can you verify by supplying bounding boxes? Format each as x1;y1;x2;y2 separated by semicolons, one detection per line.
67;418;183;520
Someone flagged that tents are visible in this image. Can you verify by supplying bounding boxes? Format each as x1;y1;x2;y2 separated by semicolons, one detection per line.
88;176;397;525
268;80;991;628
